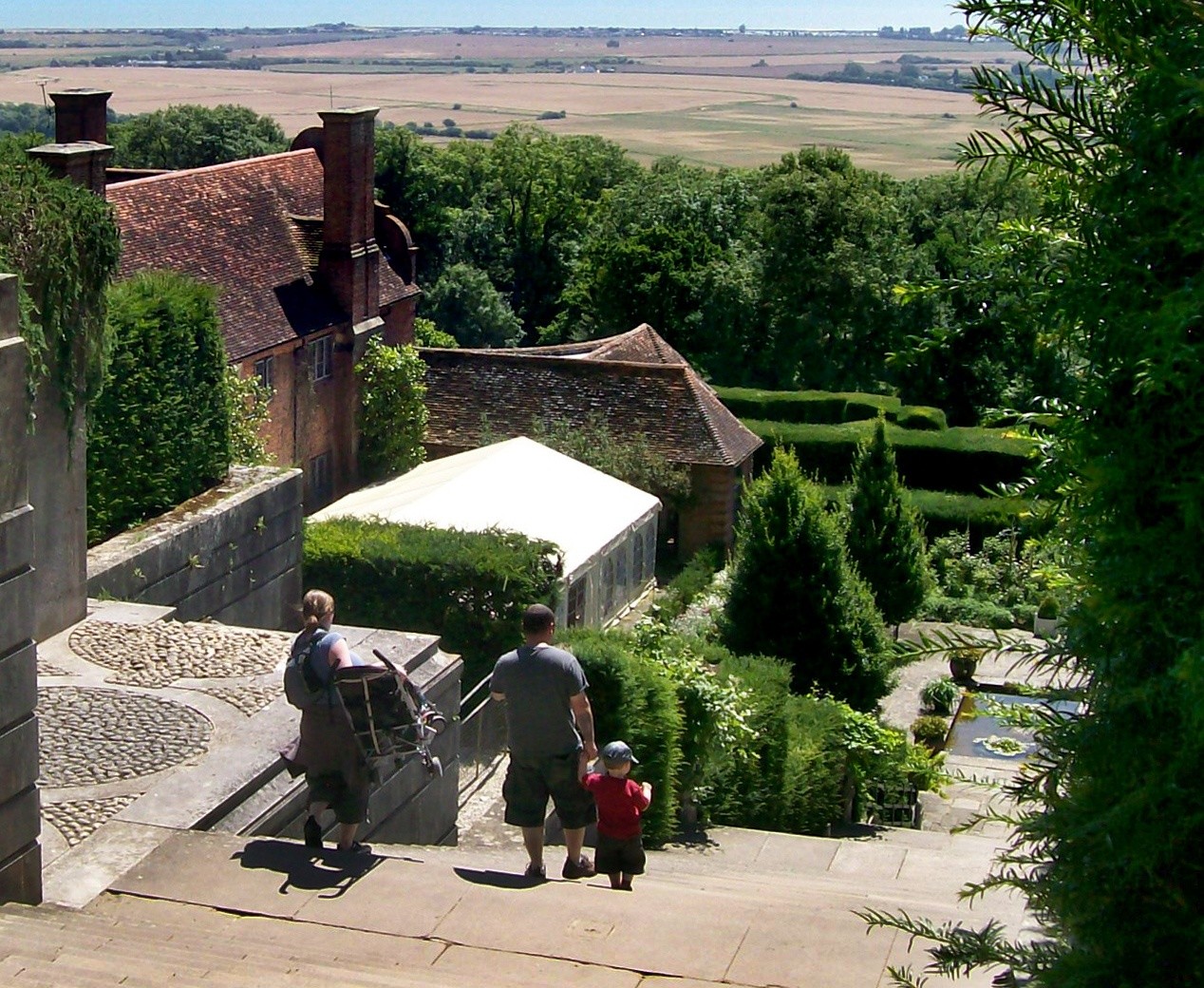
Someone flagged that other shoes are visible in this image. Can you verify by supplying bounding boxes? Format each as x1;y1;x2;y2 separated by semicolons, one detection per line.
304;816;323;846
562;854;599;879
526;862;546;878
337;840;372;855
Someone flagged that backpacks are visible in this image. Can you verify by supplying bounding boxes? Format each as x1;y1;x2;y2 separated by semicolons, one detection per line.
284;632;334;710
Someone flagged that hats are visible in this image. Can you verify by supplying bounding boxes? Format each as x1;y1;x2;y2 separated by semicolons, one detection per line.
603;741;641;766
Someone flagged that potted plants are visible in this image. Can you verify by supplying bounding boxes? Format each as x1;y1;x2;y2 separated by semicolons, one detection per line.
910;715;949;754
1034;596;1060;639
920;674;962;717
948;649;984;682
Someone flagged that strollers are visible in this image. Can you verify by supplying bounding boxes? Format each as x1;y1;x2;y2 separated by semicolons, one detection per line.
331;648;449;788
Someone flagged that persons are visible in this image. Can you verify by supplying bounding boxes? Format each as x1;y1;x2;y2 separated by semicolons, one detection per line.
578;741;652;891
284;590;406;855
489;603;598;883
